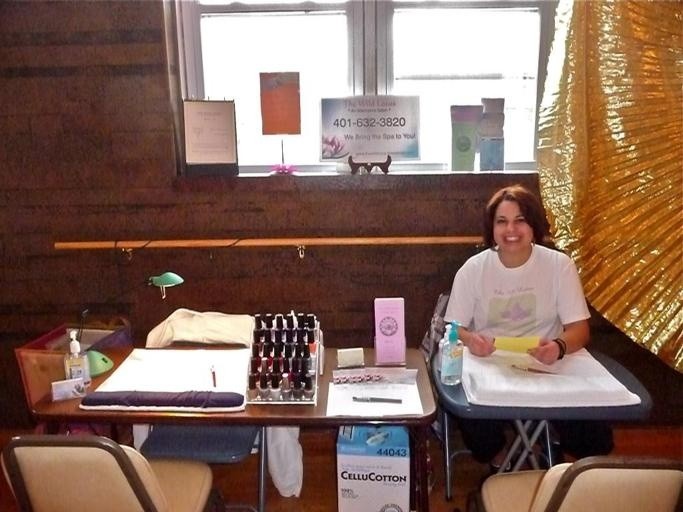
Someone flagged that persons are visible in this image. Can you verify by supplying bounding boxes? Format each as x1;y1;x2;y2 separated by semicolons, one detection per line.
443;185;615;474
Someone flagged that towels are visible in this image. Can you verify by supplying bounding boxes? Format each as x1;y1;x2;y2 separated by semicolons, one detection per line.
80;348;250;412
460;346;641;408
469;371;627;402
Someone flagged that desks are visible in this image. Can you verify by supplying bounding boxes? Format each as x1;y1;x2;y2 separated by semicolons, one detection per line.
32;347;436;512
433;349;653;471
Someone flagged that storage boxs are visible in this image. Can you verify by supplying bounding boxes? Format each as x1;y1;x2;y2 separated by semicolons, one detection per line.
337;423;411;512
15;321;131;415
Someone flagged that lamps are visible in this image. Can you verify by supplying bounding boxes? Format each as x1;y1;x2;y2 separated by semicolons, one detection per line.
76;270;184;377
259;71;302;176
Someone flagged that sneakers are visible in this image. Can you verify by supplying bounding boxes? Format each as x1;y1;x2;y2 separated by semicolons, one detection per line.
481;458;512;481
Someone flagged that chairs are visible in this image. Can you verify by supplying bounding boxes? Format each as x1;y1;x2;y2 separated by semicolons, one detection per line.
467;459;683;512
141;315;276;512
5;433;224;512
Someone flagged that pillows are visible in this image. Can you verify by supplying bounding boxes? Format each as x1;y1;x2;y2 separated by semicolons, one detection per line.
528;463;571;512
0;444;172;512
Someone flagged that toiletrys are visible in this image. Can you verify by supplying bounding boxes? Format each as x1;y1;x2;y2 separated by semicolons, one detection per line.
247;312;319;402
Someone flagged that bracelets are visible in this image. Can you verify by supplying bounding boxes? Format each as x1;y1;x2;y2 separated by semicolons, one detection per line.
553;337;566;360
